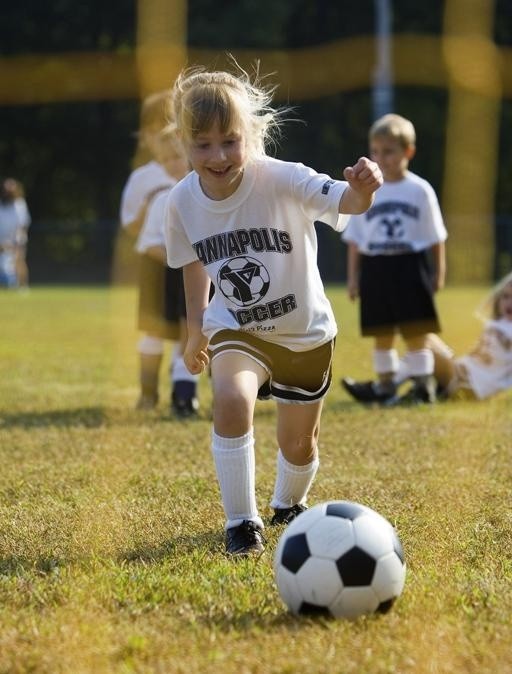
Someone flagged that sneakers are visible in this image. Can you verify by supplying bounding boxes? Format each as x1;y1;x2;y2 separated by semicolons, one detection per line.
270;503;309;527
224;518;267;556
171;381;198;419
340;376;451;407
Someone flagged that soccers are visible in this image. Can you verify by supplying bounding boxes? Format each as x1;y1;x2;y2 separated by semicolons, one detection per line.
273;500;406;624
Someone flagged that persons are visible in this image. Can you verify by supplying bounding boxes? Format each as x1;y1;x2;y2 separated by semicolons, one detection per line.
0;174;36;289
335;271;511;407
338;107;455;411
120;91;207;417
158;51;390;556
137;187;208;418
